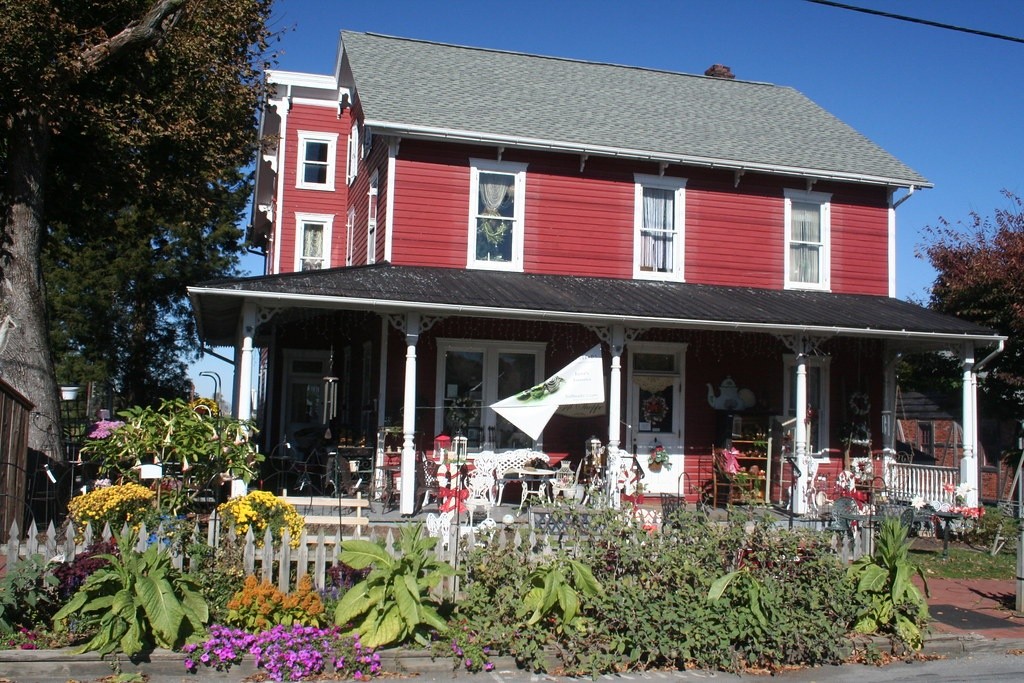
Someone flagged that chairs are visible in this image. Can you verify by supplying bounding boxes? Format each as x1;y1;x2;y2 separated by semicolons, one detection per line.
710;444;750;511
660;492;686;534
825;496;858;533
900;508;914;539
414;447;551;552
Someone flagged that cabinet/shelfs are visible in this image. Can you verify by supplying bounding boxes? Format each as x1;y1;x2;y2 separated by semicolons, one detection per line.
725;438;768;505
333;446;375;498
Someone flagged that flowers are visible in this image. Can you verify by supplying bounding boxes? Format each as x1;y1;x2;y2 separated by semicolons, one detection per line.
647;444;673;472
839;422;873;448
848;392;871;416
941;481;986;521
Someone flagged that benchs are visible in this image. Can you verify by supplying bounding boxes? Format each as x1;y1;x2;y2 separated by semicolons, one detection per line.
526;506;621;536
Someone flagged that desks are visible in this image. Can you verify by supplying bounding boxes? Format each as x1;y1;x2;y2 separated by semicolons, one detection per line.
514;467;555;518
935;511;962;557
375;466;400;515
839;513;894;556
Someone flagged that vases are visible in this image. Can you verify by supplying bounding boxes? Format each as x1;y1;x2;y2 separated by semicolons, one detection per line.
60;387;80;401
